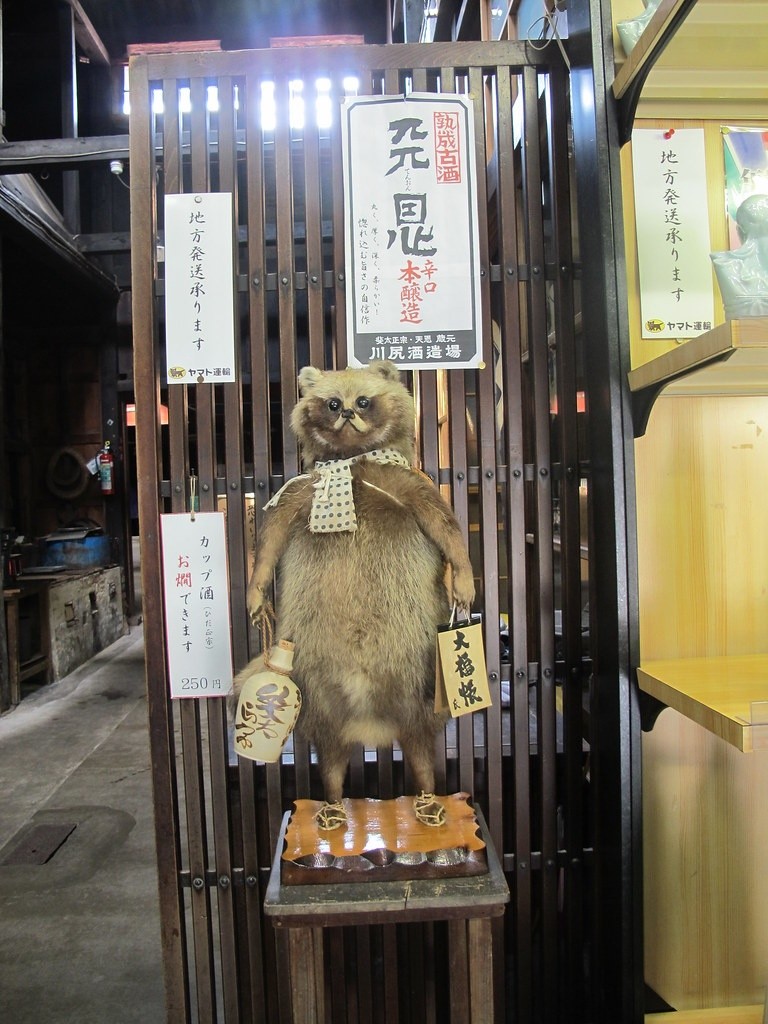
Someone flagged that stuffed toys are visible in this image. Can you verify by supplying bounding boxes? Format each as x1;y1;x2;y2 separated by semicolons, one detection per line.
246;359;475;831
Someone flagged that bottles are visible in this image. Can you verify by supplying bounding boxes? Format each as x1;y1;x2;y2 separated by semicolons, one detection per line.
231;639;303;764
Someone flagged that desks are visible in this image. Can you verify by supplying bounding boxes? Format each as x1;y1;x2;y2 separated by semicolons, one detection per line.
261;802;509;1023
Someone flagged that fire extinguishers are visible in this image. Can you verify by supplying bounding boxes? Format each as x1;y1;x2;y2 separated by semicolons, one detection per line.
95;438;116;495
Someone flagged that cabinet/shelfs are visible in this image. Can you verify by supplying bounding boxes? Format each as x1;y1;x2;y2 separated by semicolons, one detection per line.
595;0;766;1024
